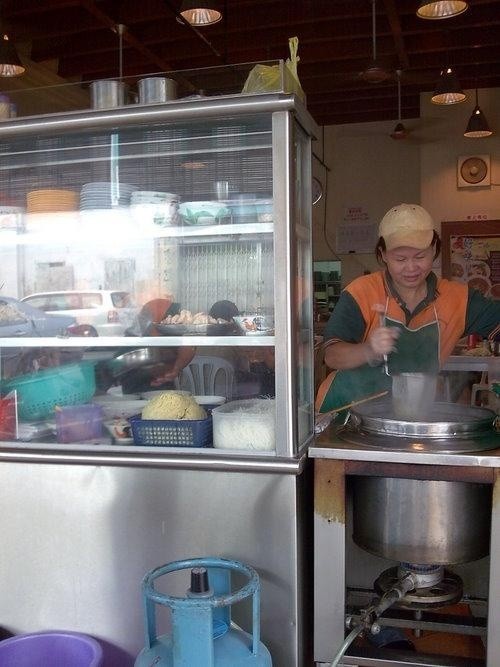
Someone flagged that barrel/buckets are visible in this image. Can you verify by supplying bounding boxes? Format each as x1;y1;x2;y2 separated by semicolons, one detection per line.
1;629;104;667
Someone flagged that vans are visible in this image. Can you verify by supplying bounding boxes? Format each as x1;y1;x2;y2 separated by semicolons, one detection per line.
19;289;138;336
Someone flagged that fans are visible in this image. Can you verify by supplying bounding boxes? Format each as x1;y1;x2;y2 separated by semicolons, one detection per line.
326;0;411;97
456;154;494;189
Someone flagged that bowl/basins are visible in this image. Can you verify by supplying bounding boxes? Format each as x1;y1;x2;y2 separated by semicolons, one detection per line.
93;390;226;442
232;315;274;335
129;191;226;230
107;347;177;377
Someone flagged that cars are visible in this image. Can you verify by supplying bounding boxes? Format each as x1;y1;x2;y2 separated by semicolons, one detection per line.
1;297;85;337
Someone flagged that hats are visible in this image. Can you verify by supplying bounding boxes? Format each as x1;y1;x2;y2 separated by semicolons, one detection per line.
377;203;442;252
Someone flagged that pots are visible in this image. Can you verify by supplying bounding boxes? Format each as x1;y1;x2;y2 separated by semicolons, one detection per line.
345;397;498;567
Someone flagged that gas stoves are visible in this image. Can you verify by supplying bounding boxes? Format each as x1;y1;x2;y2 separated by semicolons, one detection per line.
374;565;464;610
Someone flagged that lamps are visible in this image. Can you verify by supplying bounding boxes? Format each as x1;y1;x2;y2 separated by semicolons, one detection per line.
1;1;26;76
170;1;225;29
463;25;492;141
418;2;466;104
384;68;408;142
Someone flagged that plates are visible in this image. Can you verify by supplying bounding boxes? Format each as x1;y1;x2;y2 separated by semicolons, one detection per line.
25;181;139;234
151;322;235;337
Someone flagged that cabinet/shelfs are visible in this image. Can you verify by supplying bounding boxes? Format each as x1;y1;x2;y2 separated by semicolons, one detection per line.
1;88;318;667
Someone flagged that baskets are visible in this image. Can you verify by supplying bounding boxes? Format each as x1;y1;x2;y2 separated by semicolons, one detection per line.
126;408;214;447
1;361;97;420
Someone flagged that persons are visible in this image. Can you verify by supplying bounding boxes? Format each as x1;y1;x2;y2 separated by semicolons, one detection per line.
181;302;274;399
102;299;196;393
314;201;500;417
